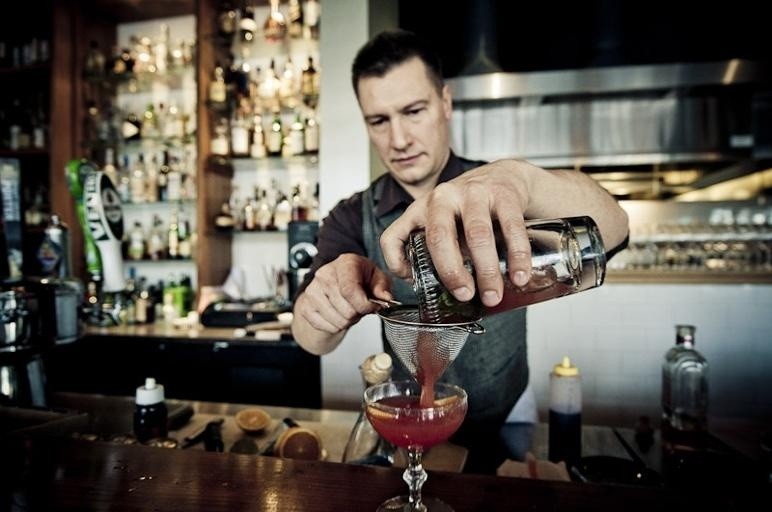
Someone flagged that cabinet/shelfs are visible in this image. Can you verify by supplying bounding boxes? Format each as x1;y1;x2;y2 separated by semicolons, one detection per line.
75;1;198;335
201;0;318;339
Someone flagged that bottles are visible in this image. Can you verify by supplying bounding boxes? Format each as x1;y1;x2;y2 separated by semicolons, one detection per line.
96;28;199;326
214;178;320;232
549;352;581;469
214;1;323;156
134;378;169;446
662;325;708;438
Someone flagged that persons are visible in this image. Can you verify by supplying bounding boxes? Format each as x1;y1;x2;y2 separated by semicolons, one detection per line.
291;30;630;423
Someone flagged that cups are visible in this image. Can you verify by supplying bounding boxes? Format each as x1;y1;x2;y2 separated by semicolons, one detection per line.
361;381;470;512
407;214;608;328
